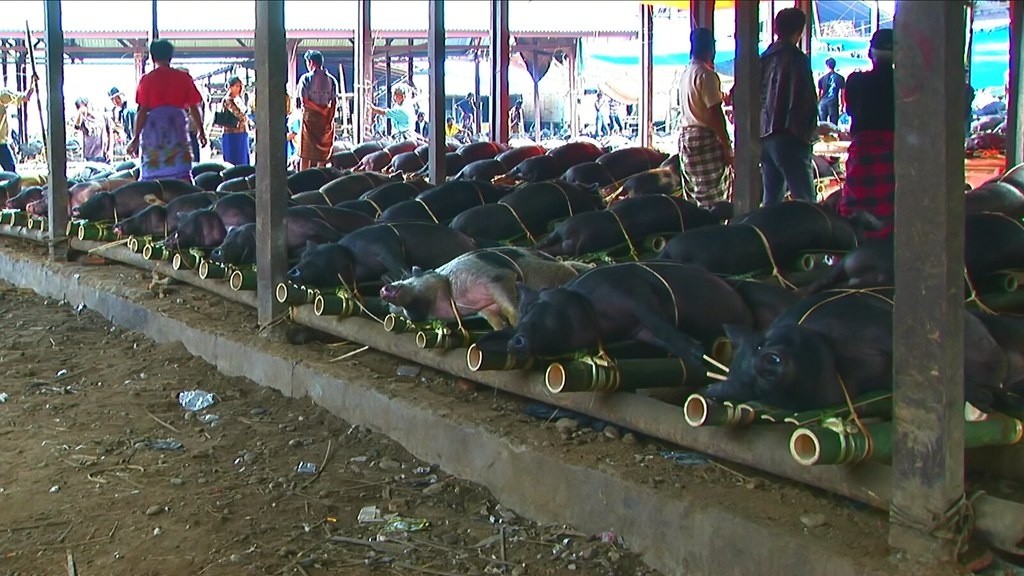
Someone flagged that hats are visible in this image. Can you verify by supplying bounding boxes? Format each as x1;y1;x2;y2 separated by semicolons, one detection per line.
868;29;893;52
108;87;125;98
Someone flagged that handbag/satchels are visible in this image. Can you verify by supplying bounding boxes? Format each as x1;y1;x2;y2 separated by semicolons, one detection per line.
214;111;240;128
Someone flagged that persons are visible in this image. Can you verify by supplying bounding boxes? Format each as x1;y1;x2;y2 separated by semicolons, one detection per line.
817;57;846;125
444;115;469;143
414;112;429;139
174;66;206;163
837;29;895;240
677;27;735;209
74;97;114;164
284;90;302;166
594;93;623;138
219;77;255;166
366;83;417;142
508;98;525;135
297;50;337;173
126;39;207;184
453;92;477;137
723;8;817;206
0;85;35;172
106;86;139;160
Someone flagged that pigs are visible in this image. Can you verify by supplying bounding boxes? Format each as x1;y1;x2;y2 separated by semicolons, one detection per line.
1;139;1024;413
